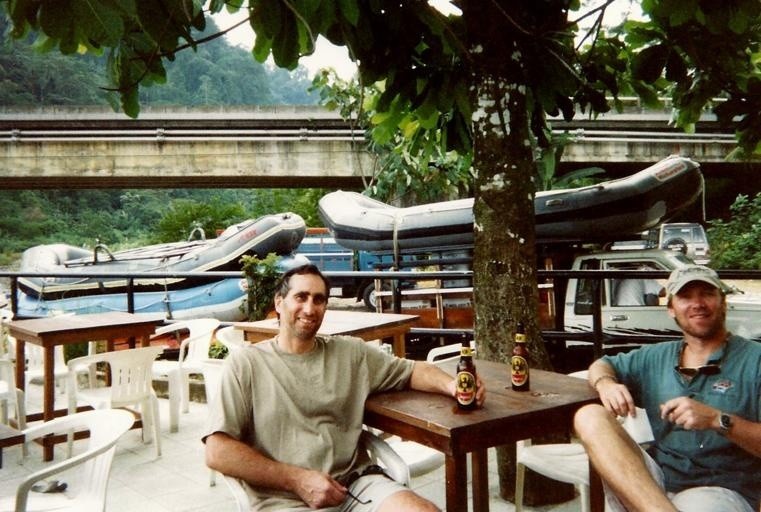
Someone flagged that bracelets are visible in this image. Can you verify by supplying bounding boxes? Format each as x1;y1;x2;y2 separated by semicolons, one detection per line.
593;375;619;391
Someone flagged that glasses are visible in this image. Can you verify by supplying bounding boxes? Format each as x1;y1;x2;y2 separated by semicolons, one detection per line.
677;339;729;377
338;464;407;504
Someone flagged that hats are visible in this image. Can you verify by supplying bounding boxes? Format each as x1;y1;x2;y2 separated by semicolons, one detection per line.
665;264;721;295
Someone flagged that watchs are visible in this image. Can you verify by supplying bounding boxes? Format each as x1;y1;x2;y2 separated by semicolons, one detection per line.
719;412;730;435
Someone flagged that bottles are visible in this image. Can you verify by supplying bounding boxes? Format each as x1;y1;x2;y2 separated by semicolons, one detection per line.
455;332;477;411
510;321;530;391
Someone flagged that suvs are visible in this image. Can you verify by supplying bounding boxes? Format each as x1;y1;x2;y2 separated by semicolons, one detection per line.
647;221;714;269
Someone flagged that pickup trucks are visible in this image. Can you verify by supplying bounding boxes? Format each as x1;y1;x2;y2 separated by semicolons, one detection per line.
368;250;761;363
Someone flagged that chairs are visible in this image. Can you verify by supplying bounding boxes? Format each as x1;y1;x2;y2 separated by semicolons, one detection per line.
220;428;411;511
0;408;136;511
216;325;249;354
54;345;170;459
7;334;97;420
149;318;221;433
515;369;654;512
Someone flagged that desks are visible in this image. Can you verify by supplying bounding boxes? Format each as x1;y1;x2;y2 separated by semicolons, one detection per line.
2;311;164;462
234;310;421;464
361;359;605;512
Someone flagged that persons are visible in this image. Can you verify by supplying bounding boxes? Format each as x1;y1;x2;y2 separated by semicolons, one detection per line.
575;264;761;512
612;267;666;307
202;265;486;512
